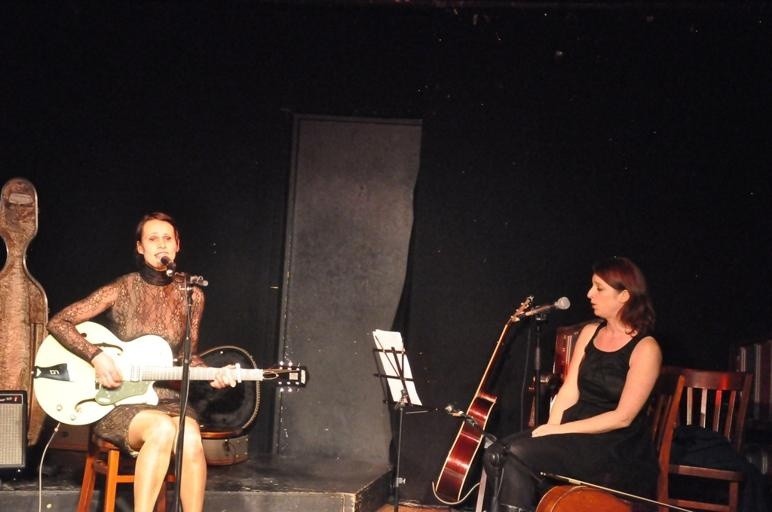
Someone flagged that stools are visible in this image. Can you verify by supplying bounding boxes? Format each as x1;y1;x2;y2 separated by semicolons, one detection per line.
76;433;177;512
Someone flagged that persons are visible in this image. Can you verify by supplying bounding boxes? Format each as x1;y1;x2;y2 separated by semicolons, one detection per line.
45;210;237;512
481;255;663;512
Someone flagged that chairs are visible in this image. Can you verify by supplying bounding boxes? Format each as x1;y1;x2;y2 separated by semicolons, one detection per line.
525;320;771;511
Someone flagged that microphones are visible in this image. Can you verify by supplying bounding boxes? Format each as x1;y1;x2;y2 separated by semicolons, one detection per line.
516;296;571;321
156;253;177;273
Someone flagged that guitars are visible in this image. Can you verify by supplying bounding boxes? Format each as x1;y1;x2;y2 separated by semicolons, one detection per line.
434;295;534;502
33;320;309;426
